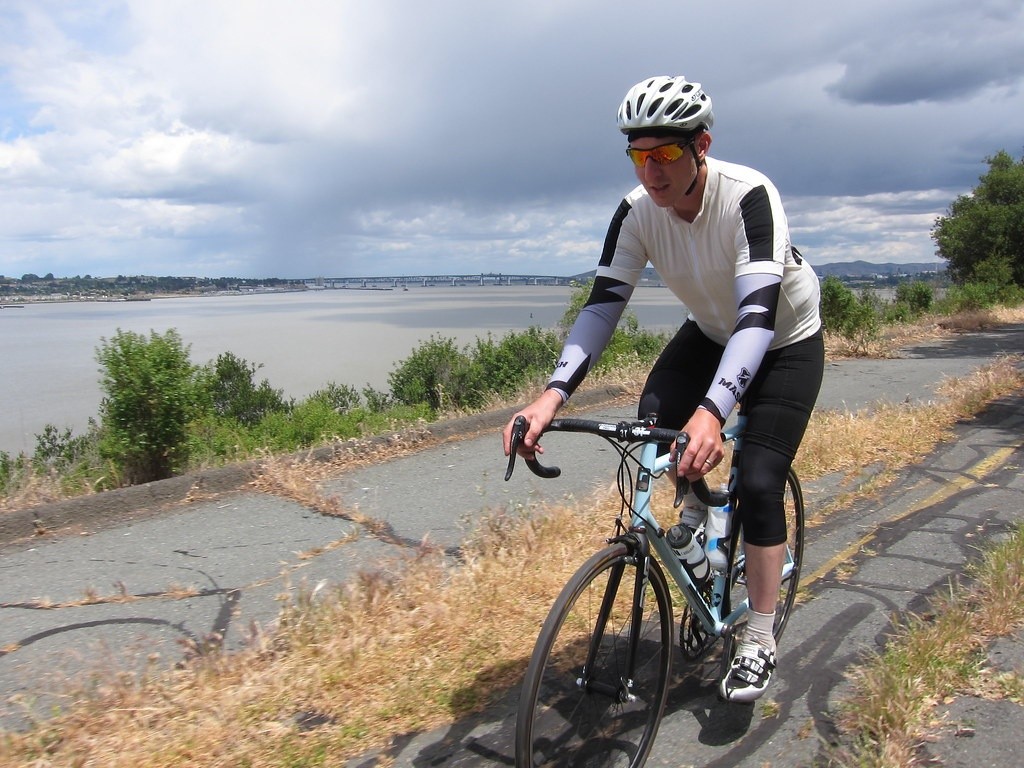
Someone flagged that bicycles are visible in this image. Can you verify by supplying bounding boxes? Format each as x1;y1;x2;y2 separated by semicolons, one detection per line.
504;414;806;768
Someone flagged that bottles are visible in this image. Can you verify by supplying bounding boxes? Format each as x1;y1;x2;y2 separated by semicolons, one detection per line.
706;482;732;567
665;524;712;581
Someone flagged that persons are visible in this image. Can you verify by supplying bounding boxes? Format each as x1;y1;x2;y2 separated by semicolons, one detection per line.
504;75;824;704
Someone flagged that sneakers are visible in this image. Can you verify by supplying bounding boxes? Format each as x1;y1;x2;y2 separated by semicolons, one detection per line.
719;634;777;704
680;506;707;545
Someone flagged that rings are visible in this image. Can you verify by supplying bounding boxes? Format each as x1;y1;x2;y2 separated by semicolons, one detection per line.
706;460;713;469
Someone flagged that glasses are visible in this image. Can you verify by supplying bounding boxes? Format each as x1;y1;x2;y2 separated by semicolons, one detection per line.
626;135;697;168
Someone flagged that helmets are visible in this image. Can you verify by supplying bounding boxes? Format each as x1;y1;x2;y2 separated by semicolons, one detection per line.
616;75;714;137
